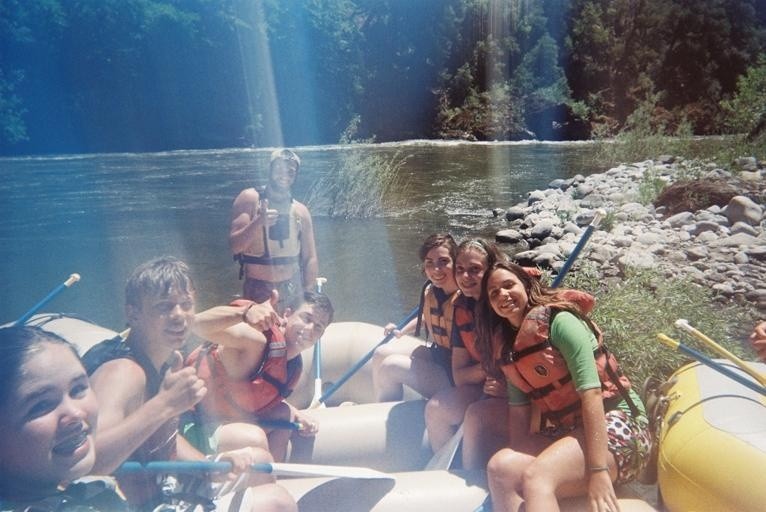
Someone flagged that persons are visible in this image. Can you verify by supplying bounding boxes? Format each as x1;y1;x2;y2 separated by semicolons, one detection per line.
748;321;766;363
423;238;542;472
80;256;278;500
371;234;461;404
0;325;298;512
474;260;653;512
180;288;334;463
230;150;319;320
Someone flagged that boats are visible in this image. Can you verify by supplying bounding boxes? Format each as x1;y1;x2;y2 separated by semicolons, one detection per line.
654;355;766;510
1;313;453;464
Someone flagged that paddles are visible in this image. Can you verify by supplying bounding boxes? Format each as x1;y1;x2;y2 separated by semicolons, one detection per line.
114;453;397;479
423;208;606;470
306;277;328;410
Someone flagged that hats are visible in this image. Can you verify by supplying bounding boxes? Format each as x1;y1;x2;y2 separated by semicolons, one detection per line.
269;147;300;168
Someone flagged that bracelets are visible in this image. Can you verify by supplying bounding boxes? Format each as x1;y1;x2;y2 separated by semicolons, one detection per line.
589;465;608;472
243;302;256;323
